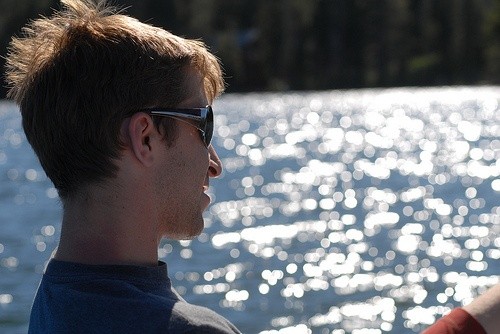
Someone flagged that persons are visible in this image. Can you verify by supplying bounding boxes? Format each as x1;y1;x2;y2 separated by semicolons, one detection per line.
0;1;500;334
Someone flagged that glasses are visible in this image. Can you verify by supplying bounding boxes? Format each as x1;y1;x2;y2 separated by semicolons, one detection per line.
112;104;216;149
